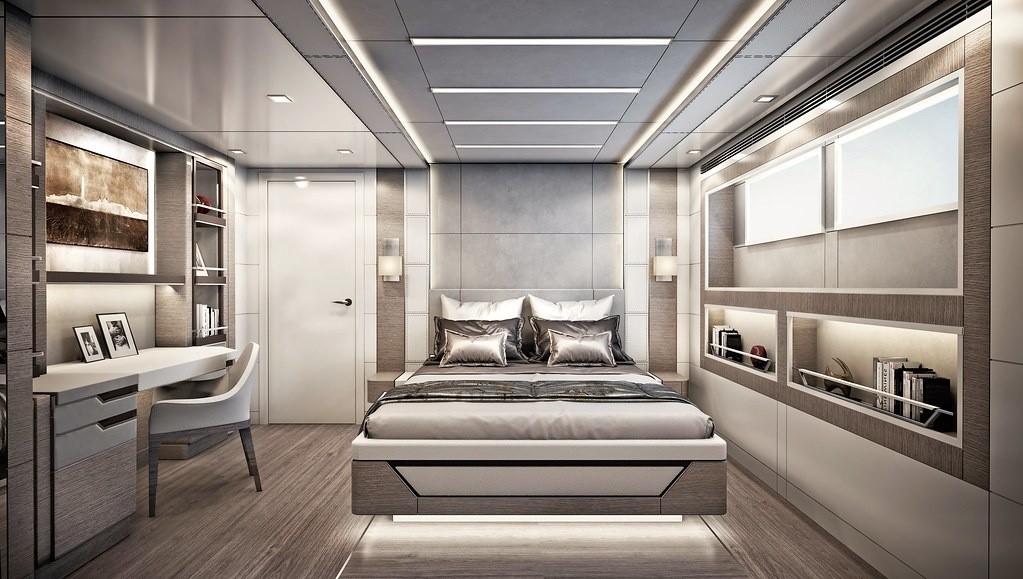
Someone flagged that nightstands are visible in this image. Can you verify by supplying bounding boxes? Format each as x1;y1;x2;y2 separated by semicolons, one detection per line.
647;372;689;398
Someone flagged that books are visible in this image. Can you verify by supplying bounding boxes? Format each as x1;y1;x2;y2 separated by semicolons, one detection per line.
197;304;220;338
713;325;741;362
873;357;950;422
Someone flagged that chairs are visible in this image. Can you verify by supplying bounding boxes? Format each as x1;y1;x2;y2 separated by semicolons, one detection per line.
147;340;260;516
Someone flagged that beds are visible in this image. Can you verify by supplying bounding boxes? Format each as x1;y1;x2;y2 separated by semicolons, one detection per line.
350;289;728;521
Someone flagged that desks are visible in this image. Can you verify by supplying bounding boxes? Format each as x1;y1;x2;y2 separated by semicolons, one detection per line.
33;346;239;406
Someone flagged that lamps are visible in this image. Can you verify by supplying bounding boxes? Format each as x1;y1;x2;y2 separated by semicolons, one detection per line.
378;237;402;282
653;237;678;282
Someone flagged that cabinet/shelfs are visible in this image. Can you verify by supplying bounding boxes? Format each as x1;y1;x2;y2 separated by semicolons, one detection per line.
51;392;137;560
156;154;229;347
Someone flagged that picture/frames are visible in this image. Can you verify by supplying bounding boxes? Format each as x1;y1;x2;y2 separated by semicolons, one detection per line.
44;136;149;253
96;312;139;359
72;324;105;363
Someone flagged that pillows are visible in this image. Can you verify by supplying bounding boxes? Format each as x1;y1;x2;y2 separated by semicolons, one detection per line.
440;293;526;321
439;328;508;369
422;316;530;365
527;316;636;364
528;294;616;321
547;328;617;367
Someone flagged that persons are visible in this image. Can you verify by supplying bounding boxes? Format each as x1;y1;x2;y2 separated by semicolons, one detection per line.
109;321;129;350
